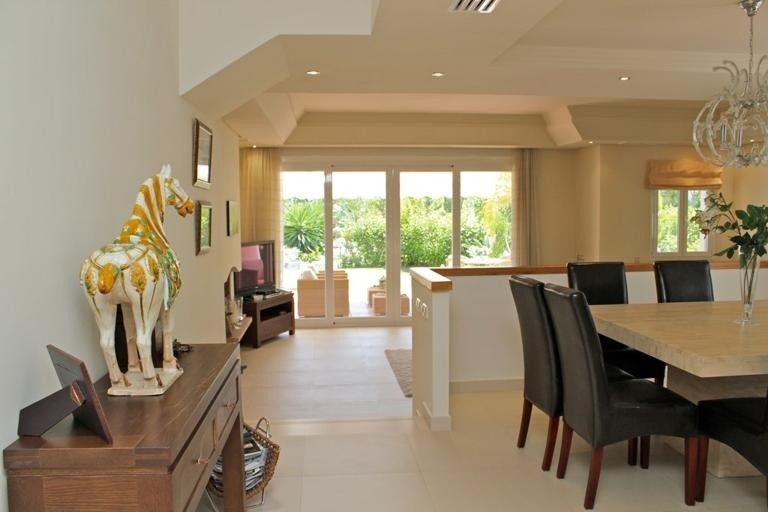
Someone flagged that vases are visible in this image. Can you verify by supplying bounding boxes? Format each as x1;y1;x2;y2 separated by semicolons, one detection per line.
732;244;762;328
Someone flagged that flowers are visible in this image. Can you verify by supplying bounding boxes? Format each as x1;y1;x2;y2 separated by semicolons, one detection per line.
688;186;768;304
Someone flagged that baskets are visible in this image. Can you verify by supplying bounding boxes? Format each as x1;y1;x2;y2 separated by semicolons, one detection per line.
205;417;280;501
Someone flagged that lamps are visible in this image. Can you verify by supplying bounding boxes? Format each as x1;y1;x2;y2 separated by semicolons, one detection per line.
688;0;768;171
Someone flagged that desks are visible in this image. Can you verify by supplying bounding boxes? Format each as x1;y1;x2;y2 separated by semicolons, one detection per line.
227;315;252;375
2;341;250;510
240;288;296;348
587;296;767;481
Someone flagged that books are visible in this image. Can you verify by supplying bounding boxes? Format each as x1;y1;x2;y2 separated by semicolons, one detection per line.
209;422;265;492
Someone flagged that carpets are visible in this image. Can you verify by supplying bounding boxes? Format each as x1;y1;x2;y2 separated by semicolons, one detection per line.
384;346;413;398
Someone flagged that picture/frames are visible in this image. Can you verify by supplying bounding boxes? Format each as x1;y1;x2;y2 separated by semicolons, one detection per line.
192;117;217;190
194;199;214;257
14;343;118;447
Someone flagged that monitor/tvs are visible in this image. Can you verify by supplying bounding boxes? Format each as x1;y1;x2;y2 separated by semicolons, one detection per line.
234;240;276;296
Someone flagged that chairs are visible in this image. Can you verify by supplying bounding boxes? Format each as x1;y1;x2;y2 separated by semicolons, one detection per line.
296;265;410;318
566;260;668;387
508;271;638;471
652;257;716;303
540;282;698;510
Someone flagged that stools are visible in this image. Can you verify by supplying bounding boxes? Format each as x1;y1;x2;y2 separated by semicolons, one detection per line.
696;388;767;503
